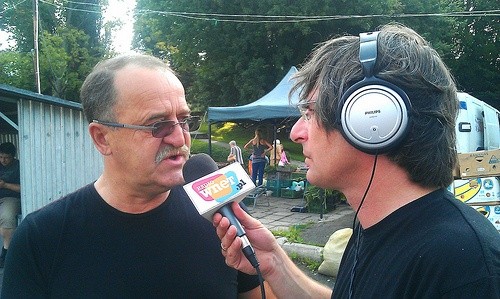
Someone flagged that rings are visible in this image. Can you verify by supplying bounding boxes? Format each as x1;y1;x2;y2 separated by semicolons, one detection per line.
219;242;226;251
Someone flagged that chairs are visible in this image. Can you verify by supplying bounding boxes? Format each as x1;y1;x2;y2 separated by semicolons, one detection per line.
242;185;274;211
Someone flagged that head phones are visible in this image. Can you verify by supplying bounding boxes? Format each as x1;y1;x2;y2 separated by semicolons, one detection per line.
336;31;414;155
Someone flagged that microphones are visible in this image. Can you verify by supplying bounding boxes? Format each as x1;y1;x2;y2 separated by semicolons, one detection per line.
182;153;260;267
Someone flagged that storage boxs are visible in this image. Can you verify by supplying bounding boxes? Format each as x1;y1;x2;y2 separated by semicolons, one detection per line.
447;148;500;234
266;172;308;199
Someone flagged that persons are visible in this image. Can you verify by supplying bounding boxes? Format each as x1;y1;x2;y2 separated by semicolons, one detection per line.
0;52;279;299
227;140;244;167
0;142;21;269
243;128;290;187
211;23;500;299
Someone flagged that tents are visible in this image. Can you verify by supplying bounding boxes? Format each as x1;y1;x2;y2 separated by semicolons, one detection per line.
206;65;324;218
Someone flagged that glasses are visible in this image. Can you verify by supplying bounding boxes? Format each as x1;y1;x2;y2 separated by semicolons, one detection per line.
89;114;202;140
296;100;316;120
